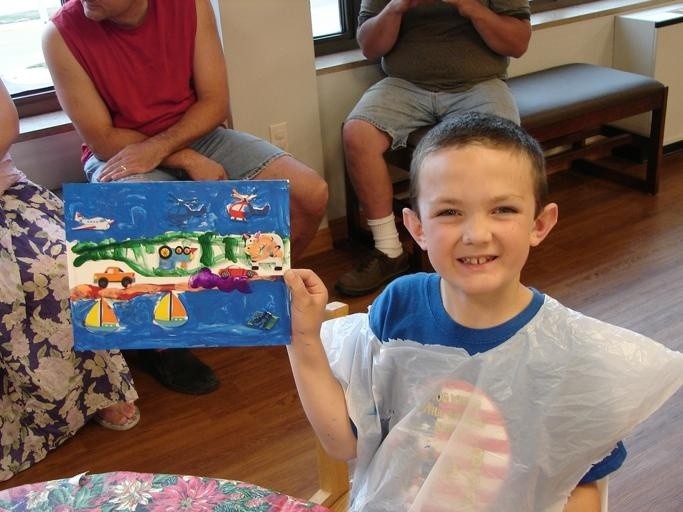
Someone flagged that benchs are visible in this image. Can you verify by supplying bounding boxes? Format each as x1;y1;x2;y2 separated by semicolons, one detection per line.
341;62;669;273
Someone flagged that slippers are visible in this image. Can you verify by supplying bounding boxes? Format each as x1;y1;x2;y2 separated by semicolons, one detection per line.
91;402;141;431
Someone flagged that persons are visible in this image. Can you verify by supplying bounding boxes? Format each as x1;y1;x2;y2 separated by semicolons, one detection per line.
282;112;625;512
41;1;331;393
336;1;533;297
1;76;140;485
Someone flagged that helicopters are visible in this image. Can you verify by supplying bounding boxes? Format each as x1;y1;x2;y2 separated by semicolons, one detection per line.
226;189;270;221
167;192;205;226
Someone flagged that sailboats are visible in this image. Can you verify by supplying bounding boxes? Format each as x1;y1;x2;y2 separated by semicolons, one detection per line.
152;291;188;329
83;296;120;335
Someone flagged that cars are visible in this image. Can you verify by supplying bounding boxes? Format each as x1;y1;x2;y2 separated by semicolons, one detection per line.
218;264;258;279
93;266;135;287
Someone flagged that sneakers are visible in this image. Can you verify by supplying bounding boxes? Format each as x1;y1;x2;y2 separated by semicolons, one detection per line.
334;249;408;294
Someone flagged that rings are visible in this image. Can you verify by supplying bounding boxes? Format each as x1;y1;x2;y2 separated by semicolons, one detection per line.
121;165;127;171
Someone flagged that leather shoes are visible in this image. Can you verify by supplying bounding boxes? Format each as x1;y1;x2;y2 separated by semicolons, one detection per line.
135;346;221;395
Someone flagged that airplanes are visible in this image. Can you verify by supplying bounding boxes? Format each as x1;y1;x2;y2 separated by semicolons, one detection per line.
71;211;114;231
